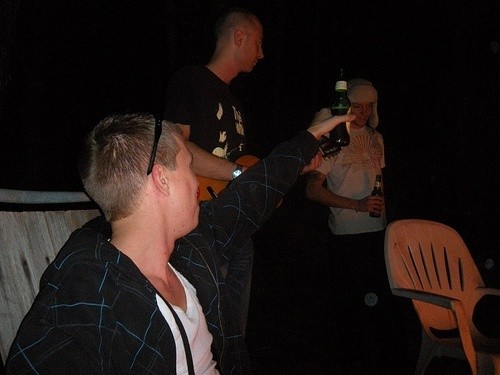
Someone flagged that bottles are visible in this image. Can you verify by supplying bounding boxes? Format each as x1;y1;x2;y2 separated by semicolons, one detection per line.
331;65;352;147
369;174;384;218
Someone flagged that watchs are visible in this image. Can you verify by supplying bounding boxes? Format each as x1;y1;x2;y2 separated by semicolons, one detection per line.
232;164;242;180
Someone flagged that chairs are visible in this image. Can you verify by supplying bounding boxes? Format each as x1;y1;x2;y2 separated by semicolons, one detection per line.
385;218;500;375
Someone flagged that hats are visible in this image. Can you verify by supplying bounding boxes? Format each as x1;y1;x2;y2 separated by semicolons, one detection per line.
346;79;379;130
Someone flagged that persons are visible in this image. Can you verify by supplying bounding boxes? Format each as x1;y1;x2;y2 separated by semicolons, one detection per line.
3;112;356;375
176;12;264;339
301;79;387;259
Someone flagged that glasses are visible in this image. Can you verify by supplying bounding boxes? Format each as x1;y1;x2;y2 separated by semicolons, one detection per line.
146;113;165;175
